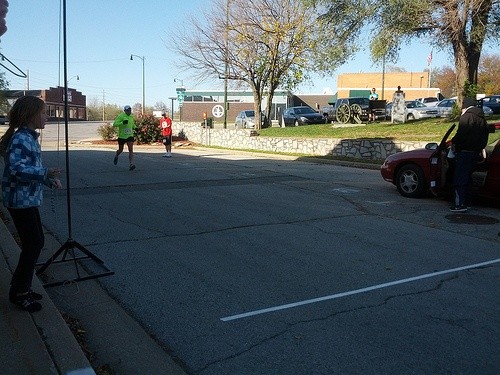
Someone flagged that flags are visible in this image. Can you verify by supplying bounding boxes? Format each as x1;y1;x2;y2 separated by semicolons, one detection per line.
427;50;432;66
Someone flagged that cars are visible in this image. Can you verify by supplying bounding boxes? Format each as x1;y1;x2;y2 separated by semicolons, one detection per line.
322;94;500;122
279;107;326;128
381;123;500;199
235;110;272;129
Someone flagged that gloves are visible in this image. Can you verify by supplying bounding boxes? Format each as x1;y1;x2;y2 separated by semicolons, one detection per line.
135;128;138;132
124;120;128;124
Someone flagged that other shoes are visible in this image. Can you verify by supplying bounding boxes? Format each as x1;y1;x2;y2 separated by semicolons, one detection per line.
8;292;42;311
113;156;118;165
164;152;171;157
129;164;136;171
32;292;43;299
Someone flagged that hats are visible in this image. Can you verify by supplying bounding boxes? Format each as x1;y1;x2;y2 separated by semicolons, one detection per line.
461;97;475;110
162;112;166;116
124;106;131;111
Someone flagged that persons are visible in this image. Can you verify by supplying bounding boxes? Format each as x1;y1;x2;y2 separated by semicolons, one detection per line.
112;105;140;170
446;99;489;212
159;112;172;156
0;96;63;312
369;87;378;124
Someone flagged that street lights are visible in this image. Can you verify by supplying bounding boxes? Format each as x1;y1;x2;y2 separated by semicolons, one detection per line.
130;54;145;113
62;75;79;118
174;78;183;121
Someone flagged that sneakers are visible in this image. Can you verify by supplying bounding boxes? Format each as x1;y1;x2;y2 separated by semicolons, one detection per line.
450;205;468;212
